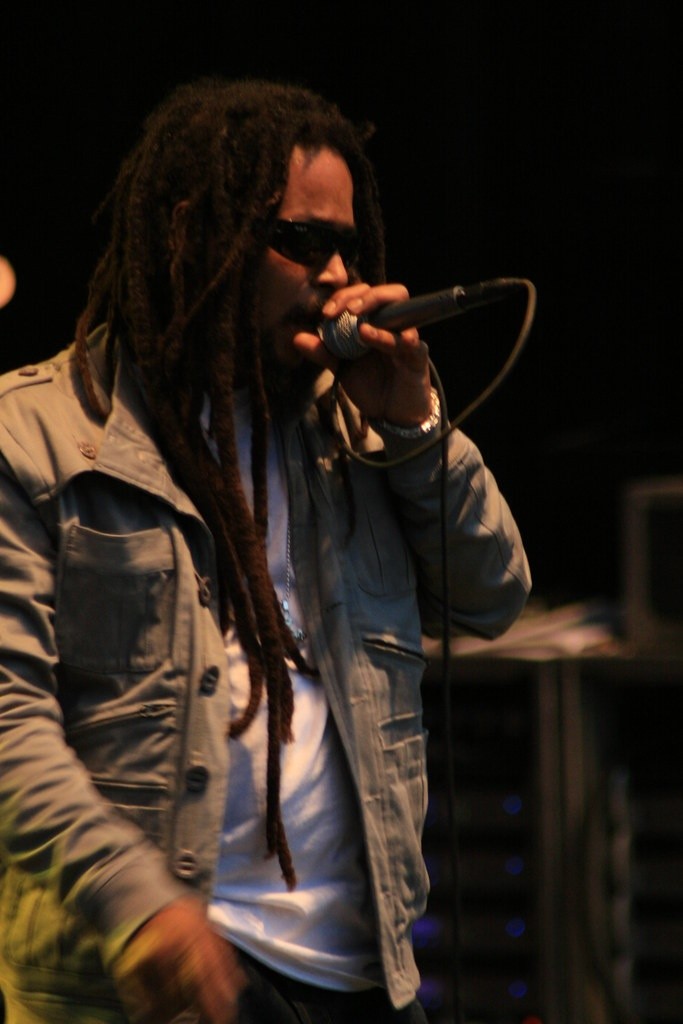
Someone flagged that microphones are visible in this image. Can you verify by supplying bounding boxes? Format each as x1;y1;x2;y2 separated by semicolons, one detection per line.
321;277;523;360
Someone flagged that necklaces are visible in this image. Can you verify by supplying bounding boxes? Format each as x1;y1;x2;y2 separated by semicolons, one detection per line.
270;518;306;641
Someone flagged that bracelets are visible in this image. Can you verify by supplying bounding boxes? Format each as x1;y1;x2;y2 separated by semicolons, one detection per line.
377;388;442;439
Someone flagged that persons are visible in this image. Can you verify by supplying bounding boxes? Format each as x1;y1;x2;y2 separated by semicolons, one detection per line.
0;85;534;1024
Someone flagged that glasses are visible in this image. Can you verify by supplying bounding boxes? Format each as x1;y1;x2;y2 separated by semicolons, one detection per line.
272;218;367;271
